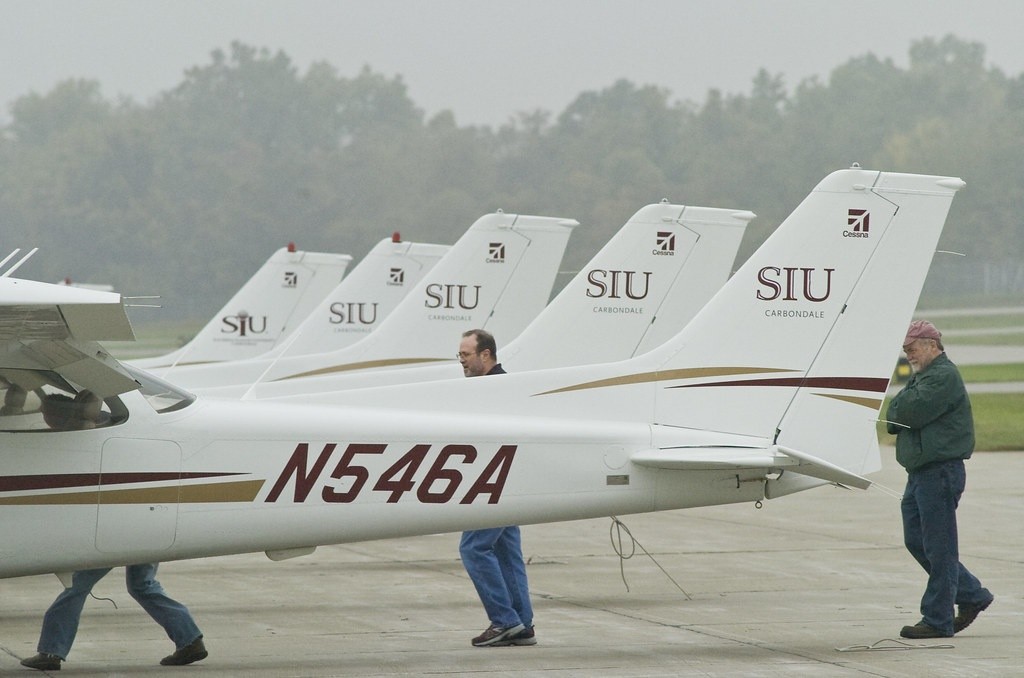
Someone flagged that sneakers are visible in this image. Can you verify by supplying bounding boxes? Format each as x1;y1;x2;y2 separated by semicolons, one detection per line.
899;620;955;638
470;621;526;647
954;588;995;633
489;625;536;646
160;638;207;666
20;653;63;671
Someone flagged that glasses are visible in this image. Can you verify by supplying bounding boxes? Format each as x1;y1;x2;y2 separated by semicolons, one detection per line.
456;346;488;359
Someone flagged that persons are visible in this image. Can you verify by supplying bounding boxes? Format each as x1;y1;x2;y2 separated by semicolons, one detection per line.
884;321;994;639
18;394;210;672
457;329;535;646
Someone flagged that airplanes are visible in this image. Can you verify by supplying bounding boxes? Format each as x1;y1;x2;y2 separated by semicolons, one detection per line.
0;161;967;582
146;208;581;391
190;195;759;401
250;231;454;363
119;240;355;371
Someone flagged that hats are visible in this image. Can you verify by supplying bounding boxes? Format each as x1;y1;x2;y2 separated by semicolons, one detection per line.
901;320;943;347
